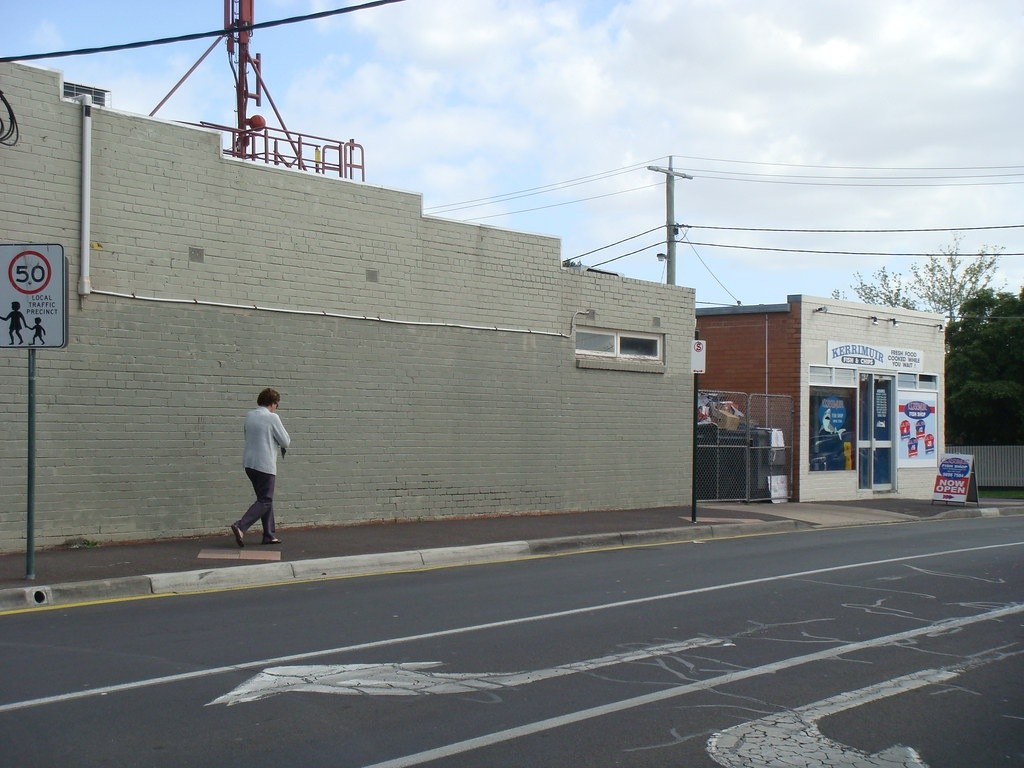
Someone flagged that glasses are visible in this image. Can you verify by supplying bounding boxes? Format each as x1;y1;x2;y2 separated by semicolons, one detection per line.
276;404;278;409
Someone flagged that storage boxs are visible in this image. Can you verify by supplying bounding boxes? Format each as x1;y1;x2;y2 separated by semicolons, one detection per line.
711;401;744;429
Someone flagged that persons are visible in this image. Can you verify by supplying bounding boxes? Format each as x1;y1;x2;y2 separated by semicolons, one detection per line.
230;389;291;546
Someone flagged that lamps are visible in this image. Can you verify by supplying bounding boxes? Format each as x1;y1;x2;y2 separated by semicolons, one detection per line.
816;307;827;313
890;318;898;327
937;324;944;332
871;316;878;324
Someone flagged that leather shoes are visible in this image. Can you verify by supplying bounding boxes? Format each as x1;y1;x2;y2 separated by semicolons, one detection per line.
231;524;244;547
262;538;282;544
